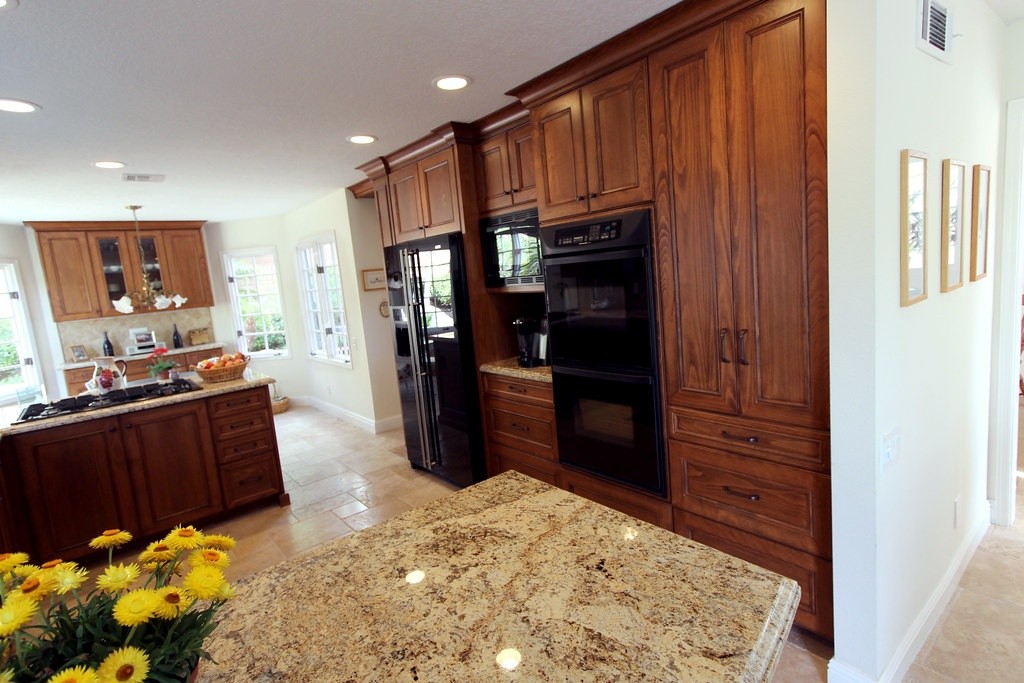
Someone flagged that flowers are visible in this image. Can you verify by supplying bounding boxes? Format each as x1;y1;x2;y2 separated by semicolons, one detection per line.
0;523;240;683
146;348;183;378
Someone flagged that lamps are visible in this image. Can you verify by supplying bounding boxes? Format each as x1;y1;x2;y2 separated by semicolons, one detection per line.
109;202;189;314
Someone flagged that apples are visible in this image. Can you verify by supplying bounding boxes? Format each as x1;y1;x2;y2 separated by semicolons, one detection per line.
201;352;244;370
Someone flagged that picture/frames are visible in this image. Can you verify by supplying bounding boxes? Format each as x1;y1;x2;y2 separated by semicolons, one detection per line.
361;268;387;291
899;148;929;306
970;163;991;283
941;157;964;294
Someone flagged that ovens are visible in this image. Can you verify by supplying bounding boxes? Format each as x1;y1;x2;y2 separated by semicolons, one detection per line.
539;208;669;498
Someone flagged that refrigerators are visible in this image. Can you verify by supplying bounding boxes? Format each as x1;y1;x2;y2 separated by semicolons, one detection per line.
383;231;486;487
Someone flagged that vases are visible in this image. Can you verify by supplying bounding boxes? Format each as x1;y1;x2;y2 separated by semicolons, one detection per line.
53;619;199;683
159;367;171;381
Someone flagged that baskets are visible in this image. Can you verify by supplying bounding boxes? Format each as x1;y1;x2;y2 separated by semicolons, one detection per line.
269;383;289;414
189;355;251;382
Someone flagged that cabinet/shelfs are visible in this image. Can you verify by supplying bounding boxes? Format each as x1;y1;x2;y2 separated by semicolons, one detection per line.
63;347;220;392
12;384;287;569
353;124;464;250
470;0;832;640
31;228;215;323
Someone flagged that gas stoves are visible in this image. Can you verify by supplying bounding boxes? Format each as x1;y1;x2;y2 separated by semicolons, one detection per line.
10;378;203;425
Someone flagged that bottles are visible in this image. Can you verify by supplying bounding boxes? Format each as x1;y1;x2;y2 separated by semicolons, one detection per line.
103;331;114;357
173;324;184;348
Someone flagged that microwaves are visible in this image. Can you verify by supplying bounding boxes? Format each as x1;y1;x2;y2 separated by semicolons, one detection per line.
479;206;545;289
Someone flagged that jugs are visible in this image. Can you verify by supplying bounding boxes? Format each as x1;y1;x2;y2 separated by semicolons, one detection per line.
90;357;127;395
